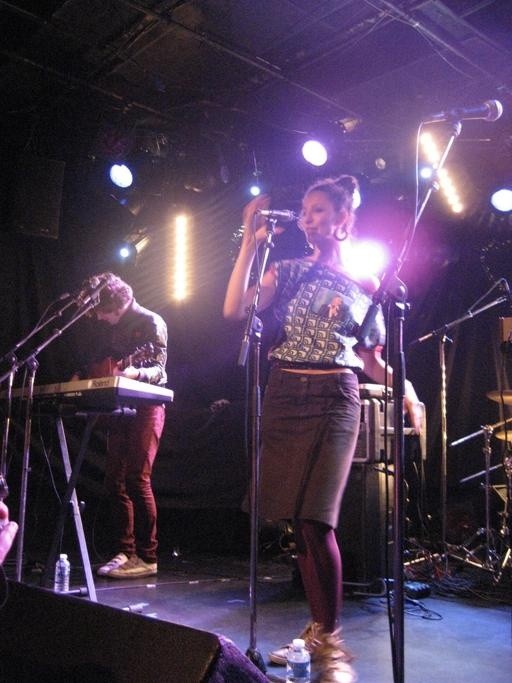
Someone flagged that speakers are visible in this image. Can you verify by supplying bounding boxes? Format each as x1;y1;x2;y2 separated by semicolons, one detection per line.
10;158;65;239
296;463;394;596
0;576;274;683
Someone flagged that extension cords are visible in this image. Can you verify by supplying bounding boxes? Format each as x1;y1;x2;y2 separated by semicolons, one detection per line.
406;584;431;598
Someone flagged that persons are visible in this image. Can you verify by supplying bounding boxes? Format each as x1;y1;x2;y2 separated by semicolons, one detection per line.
68;272;169;580
221;172;425;682
0;475;17;571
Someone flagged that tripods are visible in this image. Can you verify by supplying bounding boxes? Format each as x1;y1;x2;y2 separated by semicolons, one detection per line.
403;332;498;573
457;432;512;566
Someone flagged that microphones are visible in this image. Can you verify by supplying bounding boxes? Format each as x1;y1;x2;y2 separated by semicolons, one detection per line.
430;100;502;123
500;279;512;308
258;209;296;222
49;292;70;306
76;278;100;304
84;278;109;304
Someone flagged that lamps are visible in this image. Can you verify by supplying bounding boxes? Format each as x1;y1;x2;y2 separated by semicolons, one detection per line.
106;134;169;191
116;222;155;261
299;112;343;169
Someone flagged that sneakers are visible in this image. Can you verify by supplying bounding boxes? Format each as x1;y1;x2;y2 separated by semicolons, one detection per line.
312;626;358;683
267;623;316;664
97;552;158;578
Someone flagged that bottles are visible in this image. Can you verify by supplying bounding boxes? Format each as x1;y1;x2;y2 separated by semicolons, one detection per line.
54;553;70;594
284;637;312;681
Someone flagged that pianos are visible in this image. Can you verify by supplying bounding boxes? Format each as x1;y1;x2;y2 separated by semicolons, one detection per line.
0;375;174;410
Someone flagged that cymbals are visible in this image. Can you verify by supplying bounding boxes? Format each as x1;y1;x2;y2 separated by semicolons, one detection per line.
485;389;512;405
495;430;511;443
